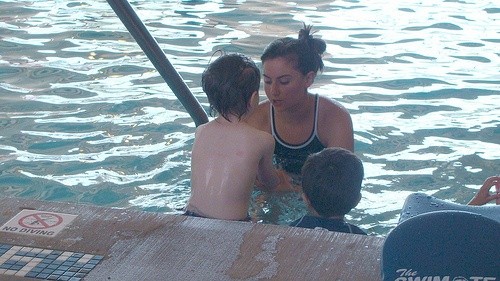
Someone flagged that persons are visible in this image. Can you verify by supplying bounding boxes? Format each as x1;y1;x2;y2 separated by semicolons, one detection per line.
466;176;500;206
242;28;354;195
184;54;281;221
288;146;368;236
